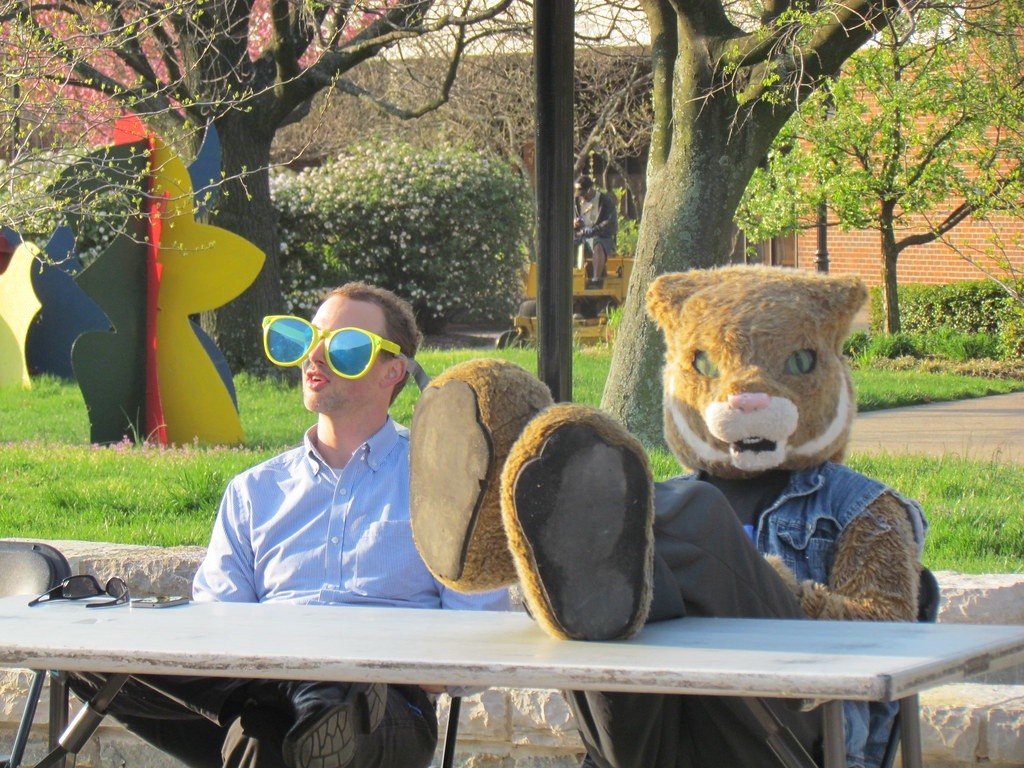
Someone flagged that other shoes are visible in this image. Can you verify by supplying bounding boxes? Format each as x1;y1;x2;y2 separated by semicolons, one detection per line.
587;278;602;289
283;678;388;768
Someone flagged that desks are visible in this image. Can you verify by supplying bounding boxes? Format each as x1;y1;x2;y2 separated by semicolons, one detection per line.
1;594;1024;768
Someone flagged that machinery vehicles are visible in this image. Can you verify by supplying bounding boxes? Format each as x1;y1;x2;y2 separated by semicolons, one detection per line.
491;242;637;354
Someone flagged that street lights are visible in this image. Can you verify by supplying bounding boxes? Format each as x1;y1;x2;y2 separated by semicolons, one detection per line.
812;68;841;275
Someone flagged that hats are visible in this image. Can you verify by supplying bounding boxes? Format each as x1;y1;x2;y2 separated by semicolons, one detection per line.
573;176;593;197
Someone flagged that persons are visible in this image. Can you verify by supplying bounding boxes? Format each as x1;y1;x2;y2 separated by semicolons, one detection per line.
68;281;509;767
574;173;618;290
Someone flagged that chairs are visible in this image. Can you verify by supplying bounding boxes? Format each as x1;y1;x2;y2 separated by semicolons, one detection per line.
1;541;73;768
579;564;940;768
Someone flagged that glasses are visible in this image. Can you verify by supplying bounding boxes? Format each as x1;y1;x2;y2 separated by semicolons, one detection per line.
261;315;400;381
28;575;129;609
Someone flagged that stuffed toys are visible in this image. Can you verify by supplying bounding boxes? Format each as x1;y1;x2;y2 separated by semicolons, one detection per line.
411;266;920;768
